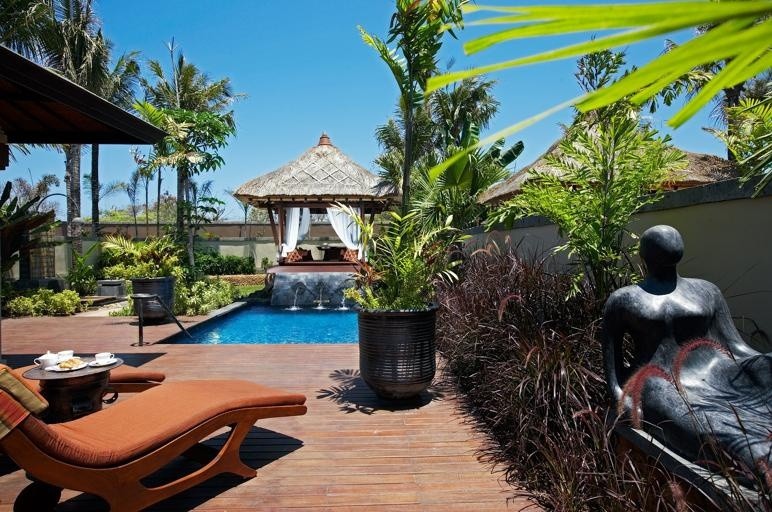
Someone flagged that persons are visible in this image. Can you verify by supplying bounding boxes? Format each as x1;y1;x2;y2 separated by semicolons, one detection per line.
596;224;772;493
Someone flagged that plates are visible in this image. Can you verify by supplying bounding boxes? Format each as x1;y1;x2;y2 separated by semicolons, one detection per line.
88;357;118;367
45;361;89;371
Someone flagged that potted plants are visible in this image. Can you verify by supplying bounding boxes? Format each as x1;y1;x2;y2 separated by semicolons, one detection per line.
101;230;187;324
331;198;475;399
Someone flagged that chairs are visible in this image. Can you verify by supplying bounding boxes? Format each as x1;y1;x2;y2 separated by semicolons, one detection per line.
1;363;306;511
1;364;166;393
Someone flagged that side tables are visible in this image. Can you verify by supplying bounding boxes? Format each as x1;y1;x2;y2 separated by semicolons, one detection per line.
23;357;122;423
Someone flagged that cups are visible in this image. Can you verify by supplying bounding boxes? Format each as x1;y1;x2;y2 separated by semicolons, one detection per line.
95;352;115;365
58;350;74;362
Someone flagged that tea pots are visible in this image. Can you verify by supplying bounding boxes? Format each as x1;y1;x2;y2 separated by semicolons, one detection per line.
33;350;63;370
